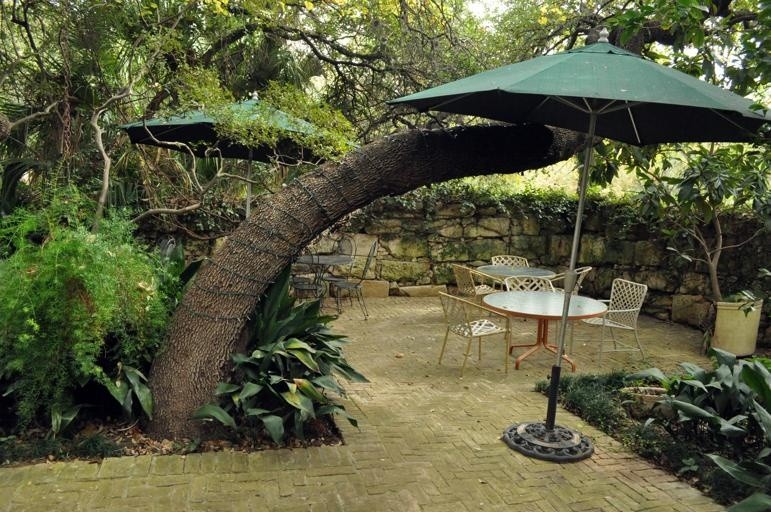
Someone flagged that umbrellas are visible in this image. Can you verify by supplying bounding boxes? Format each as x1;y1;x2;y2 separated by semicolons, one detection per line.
116;90;364;217
383;27;771;430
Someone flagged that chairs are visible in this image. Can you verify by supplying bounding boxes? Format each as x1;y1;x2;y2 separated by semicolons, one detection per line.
286;231;380;322
434;251;649;383
